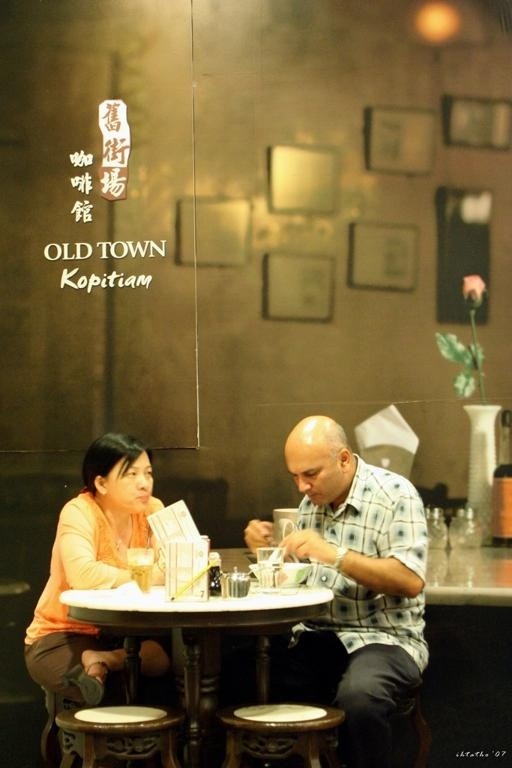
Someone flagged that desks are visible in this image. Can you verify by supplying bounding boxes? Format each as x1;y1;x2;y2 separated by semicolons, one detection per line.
399;544;512;607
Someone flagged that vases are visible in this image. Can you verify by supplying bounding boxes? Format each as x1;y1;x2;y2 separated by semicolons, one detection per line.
465;401;501;546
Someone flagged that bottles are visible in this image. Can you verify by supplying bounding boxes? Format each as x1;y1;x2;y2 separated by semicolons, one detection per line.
447;506;484;548
425;509;448;548
490;548;512;588
426;547;484;588
208;551;223;596
490;409;512;548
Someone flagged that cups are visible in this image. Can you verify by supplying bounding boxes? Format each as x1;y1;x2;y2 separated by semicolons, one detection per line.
273;507;299;537
123;548;157;592
219;572;251;598
254;544;287;589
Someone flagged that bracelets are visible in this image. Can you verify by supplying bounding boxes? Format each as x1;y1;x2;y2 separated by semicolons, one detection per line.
332;544;350;575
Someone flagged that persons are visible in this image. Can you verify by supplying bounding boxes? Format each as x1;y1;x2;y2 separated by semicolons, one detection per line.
23;430;174;707
242;414;431;768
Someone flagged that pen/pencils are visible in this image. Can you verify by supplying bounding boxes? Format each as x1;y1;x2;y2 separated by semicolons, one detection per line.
171;560;217;603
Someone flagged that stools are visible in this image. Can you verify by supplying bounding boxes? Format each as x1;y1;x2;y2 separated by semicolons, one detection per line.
54;703;187;768
216;705;346;768
396;684;432;767
41;692;79;767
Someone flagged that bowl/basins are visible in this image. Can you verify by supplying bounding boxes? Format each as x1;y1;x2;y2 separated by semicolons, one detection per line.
248;563;311;590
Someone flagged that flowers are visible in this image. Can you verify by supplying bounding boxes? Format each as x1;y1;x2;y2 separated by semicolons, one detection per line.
437;275;505;403
